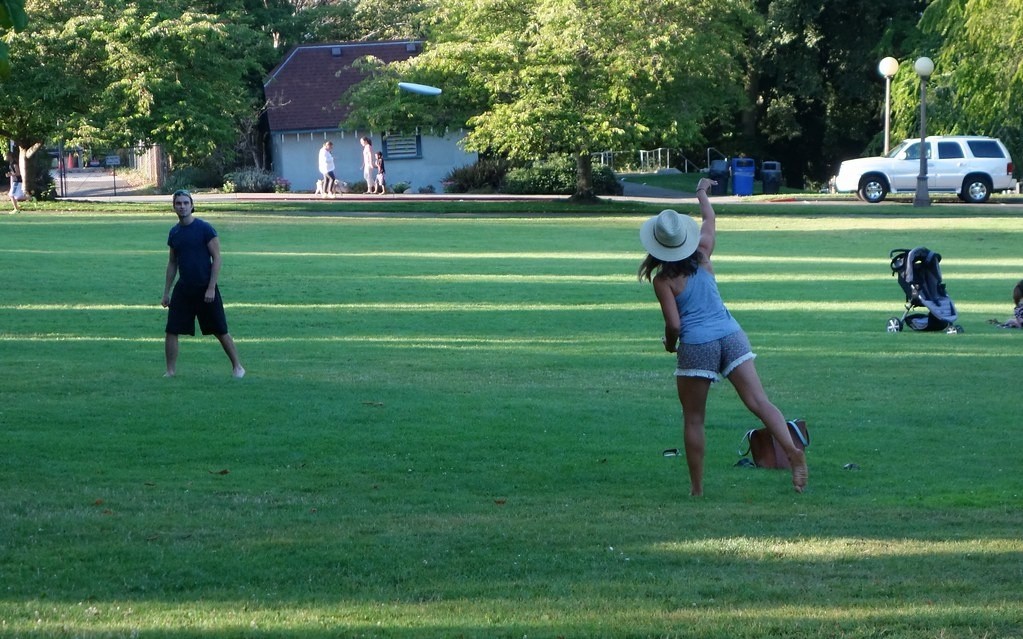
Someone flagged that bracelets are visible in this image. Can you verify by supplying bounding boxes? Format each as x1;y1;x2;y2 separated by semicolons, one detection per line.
696;188;706;192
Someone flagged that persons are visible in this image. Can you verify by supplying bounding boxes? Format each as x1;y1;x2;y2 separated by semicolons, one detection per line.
370;151;387;195
359;136;375;195
3;151;23;215
638;177;809;494
161;189;245;379
318;140;337;194
1004;279;1023;328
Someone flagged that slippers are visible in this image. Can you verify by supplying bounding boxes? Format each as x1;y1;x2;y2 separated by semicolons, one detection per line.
663;448;683;457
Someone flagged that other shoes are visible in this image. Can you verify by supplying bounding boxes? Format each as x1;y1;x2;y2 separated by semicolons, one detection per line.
734;458;754;468
842;463;862;472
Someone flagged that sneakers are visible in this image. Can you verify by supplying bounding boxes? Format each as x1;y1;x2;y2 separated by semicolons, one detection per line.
30;197;37;206
9;209;20;214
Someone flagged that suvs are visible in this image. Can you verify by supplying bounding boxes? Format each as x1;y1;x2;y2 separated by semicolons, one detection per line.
835;135;1014;204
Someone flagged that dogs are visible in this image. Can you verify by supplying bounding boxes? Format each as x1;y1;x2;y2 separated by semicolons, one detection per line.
315;179;350;196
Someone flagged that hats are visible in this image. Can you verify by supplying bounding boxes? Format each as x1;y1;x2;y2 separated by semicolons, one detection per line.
640;210;699;262
173;189;194;212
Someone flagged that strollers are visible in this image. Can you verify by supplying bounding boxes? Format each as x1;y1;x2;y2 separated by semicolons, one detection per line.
888;247;964;334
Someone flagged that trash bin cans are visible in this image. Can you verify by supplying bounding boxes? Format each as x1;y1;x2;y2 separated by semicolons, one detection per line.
708;159;729;195
761;161;781;195
729;158;754;195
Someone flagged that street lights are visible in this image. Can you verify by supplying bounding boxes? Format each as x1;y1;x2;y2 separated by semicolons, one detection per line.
878;54;900;156
914;56;936;204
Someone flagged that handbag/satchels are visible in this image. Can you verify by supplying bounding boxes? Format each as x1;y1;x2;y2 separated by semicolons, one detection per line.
738;419;811;469
